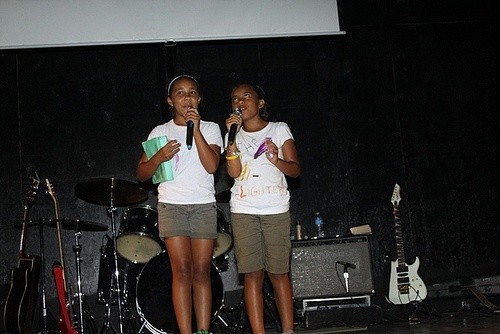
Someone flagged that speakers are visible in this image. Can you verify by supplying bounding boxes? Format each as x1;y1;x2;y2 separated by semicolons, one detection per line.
288;236;376;302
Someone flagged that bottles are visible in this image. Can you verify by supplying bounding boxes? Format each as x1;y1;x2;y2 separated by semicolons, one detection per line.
314;212;325;239
296;220;302;240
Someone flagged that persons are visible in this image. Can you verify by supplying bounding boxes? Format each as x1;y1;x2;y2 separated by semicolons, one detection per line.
136;73;224;334
224;80;302;334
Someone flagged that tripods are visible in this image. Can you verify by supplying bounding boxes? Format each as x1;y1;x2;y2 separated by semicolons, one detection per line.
69;185;140;334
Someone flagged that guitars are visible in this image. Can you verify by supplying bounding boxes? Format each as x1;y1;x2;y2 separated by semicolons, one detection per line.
387;183;428;305
4;171;41;334
46;177;79;334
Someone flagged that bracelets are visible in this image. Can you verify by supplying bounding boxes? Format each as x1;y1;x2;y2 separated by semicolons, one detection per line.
225;148;241;155
225;154;239;160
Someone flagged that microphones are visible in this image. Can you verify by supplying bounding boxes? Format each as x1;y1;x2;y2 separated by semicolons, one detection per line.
186;110;194;150
105;234;113;246
338;261;356;269
229;108;242;145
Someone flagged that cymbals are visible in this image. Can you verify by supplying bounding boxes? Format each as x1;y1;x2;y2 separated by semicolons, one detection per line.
74;178;150;208
45;219;110;233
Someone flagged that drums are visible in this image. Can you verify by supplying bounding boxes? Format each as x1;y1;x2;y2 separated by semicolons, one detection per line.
115;206;165;264
134;251;224;334
212;205;233;261
96;246;140;306
